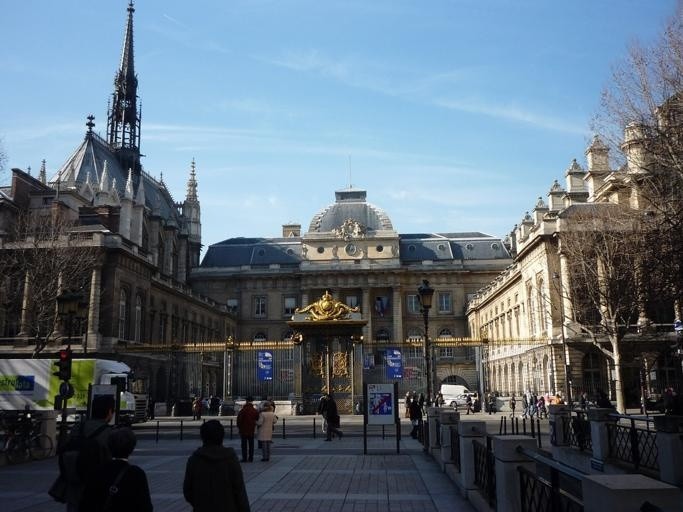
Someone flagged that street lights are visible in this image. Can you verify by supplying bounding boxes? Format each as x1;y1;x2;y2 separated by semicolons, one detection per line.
418;280;435;406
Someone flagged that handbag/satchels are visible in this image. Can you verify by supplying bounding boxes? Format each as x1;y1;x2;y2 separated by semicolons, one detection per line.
336;415;340;428
48;474;74;504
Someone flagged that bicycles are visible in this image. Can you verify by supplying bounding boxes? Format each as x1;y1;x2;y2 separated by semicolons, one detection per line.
3;413;54;466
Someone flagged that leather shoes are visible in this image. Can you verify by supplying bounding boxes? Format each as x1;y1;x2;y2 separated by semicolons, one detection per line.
324;439;331;441
339;431;343;440
239;458;253;463
261;458;269;461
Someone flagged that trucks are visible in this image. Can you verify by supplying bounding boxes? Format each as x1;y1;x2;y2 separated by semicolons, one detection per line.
0;356;151;424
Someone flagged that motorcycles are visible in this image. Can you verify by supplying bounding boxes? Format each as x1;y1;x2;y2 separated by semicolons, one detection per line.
640;395;666;414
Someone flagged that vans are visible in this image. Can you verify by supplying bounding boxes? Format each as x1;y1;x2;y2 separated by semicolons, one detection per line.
440;384;471;404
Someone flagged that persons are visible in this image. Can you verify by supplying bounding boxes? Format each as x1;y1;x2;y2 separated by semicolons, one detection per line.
318;394;343;442
183;420;251;512
405;391;442;418
580;391;586;409
466;390;562;420
207;395;214;416
192;397;202;420
409;396;422;439
58;394;153;512
236;395;278;462
593;387;620;414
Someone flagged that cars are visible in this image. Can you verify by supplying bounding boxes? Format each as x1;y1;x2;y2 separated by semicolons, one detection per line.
446;393;478;408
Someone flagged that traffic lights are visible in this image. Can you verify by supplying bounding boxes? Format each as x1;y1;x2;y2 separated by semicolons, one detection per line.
53;348;72;380
